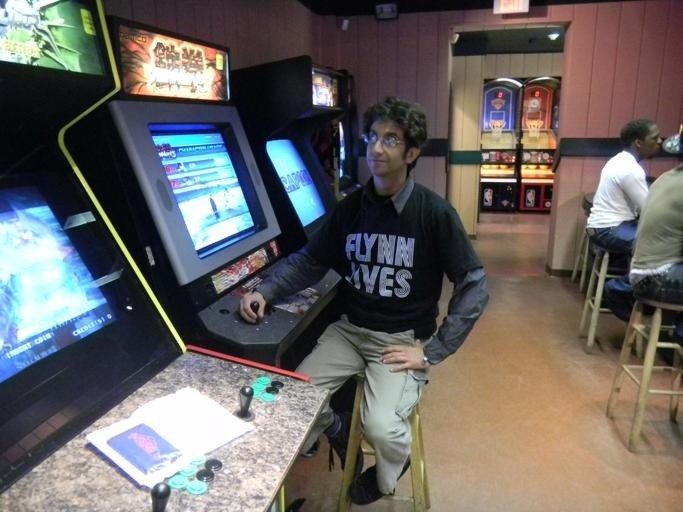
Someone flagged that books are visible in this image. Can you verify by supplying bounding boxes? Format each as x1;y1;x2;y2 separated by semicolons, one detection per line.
84;384;253;490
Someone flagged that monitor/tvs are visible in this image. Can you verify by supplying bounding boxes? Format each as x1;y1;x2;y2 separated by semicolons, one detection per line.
148;122;268;260
339;121;346;179
265;139;327;228
0;183;117;383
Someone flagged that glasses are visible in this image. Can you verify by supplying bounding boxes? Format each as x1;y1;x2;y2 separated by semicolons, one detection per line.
361;131;405;148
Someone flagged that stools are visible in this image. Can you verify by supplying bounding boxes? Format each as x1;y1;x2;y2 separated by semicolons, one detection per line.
337;373;431;511
572;191;683;452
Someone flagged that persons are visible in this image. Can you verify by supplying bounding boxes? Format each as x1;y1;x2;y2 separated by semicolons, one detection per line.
238;93;491;505
584;117;663;323
627;124;683;347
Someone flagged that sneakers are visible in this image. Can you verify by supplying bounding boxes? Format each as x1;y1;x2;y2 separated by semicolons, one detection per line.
328;411;363;478
605;291;631;322
349;456;411;505
